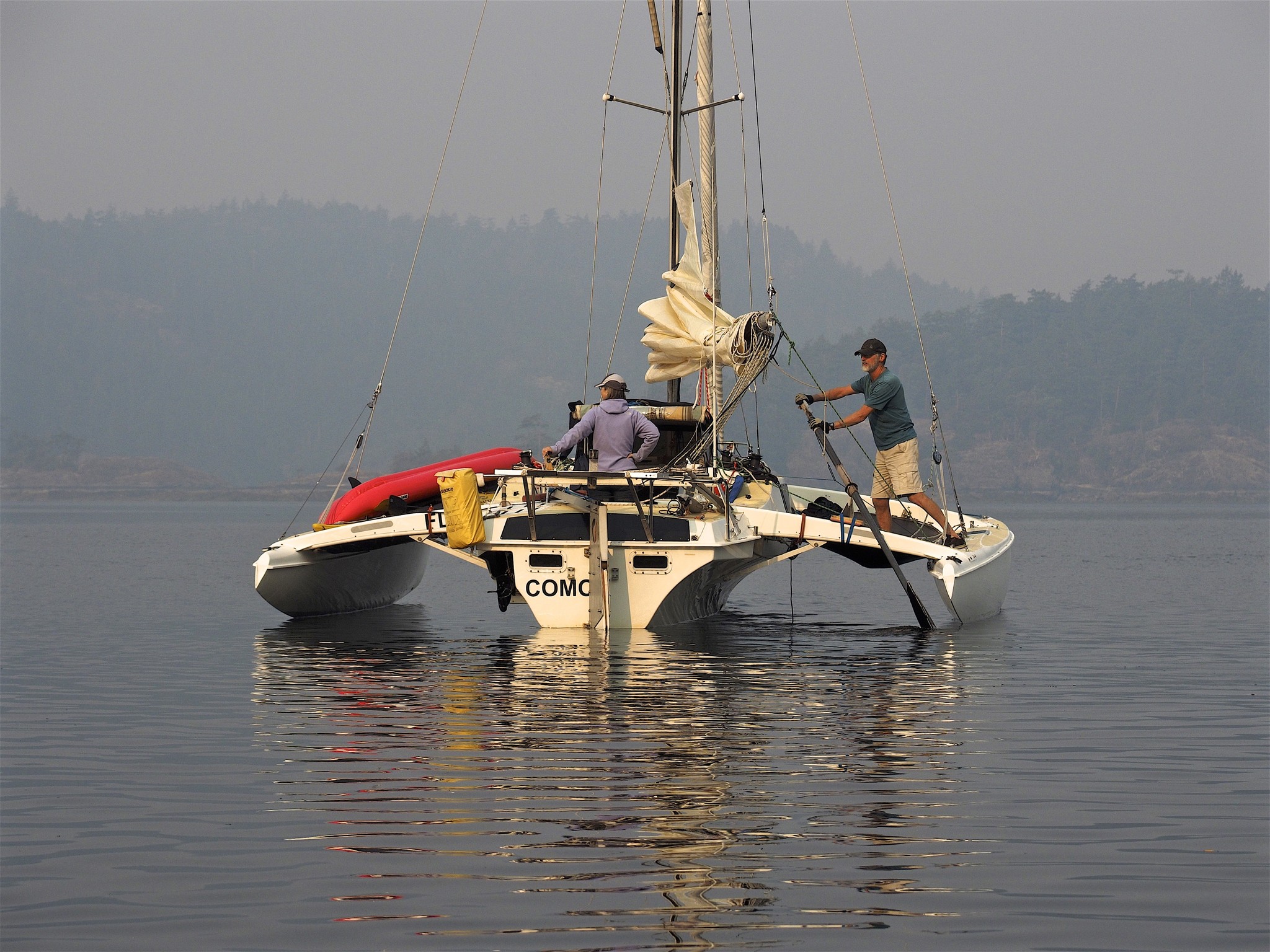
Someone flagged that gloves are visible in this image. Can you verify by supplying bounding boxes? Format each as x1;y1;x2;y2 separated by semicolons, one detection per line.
795;393;814;409
807;417;830;434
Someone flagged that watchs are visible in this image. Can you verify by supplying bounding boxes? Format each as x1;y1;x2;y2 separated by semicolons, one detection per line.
830;423;835;431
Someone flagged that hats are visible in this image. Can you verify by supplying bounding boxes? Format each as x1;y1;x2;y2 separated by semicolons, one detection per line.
854;338;887;356
594;373;630;392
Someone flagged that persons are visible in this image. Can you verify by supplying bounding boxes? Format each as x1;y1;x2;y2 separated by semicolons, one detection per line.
795;338;966;550
542;374;660;502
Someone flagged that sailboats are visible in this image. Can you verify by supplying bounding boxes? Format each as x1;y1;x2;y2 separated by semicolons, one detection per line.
248;0;1019;634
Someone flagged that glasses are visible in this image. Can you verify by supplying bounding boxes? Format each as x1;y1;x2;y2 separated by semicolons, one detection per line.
598;386;604;390
858;353;878;358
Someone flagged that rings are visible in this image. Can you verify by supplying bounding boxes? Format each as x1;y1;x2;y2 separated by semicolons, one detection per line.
543;447;545;449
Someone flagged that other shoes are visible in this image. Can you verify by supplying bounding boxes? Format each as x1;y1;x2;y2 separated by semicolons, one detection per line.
941;533;965;550
